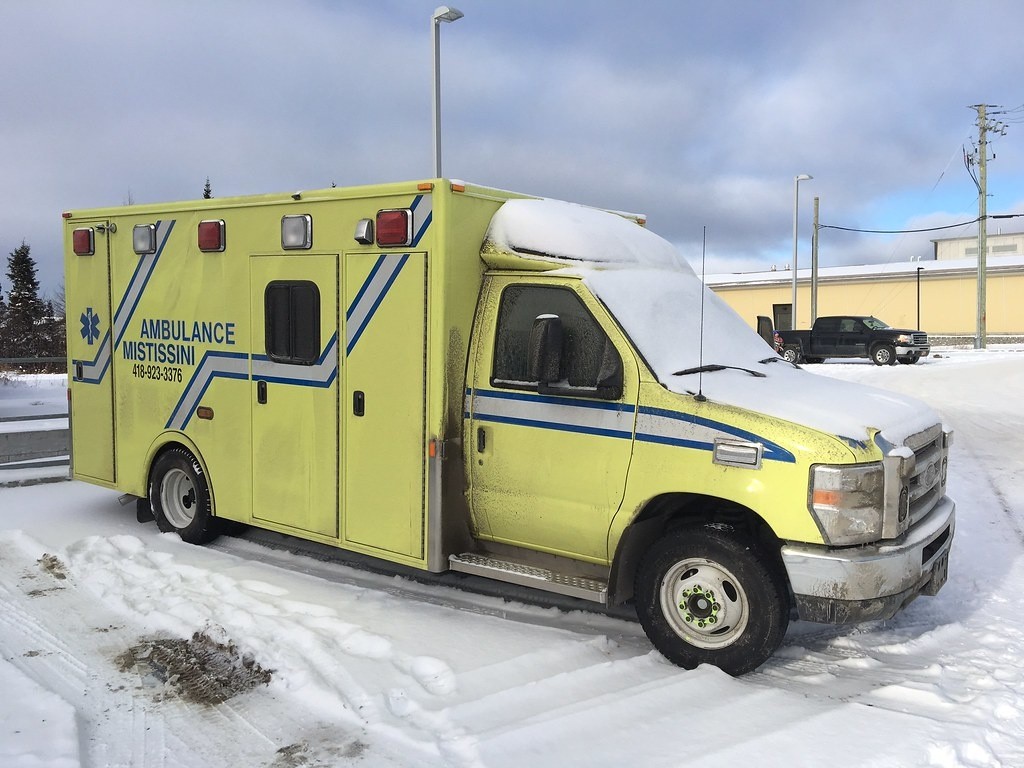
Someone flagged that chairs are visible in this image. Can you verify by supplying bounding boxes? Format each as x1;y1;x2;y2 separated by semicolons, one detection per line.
841;324;848;331
854;322;861;331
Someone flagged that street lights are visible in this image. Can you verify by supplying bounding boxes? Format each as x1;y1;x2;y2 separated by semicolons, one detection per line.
791;174;814;331
431;5;465;178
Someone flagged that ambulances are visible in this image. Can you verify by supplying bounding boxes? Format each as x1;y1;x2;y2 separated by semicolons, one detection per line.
63;177;957;677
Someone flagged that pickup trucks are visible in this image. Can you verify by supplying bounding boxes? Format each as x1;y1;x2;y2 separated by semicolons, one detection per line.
774;315;930;366
